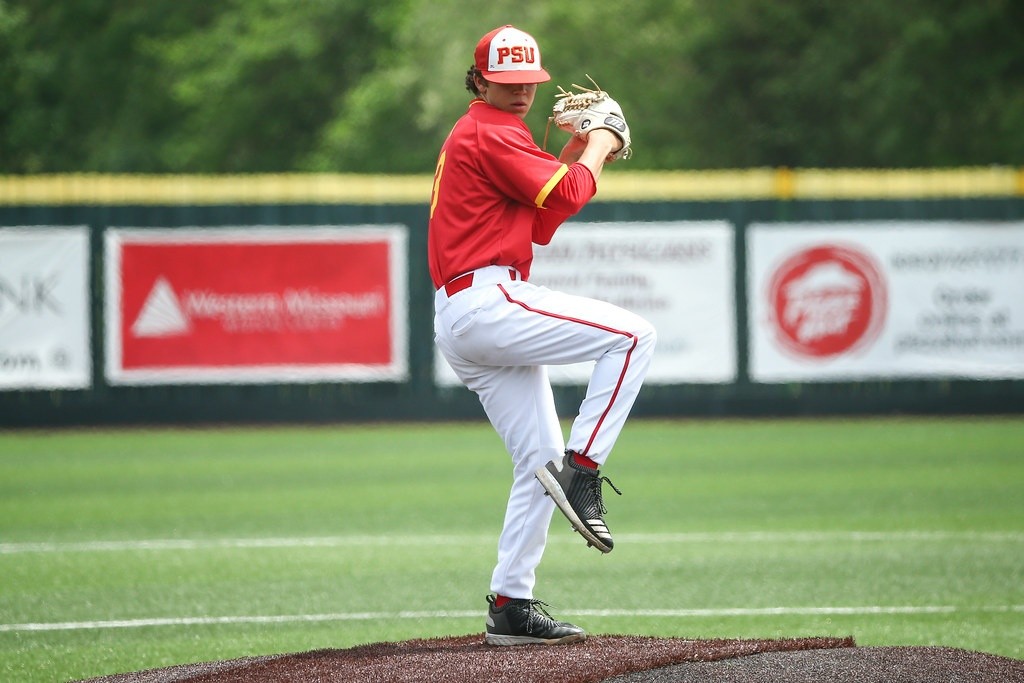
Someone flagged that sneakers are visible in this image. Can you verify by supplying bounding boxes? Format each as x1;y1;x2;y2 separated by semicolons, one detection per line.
485;593;588;645
535;451;621;553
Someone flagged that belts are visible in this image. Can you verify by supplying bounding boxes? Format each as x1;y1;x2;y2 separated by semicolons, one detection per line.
445;268;516;296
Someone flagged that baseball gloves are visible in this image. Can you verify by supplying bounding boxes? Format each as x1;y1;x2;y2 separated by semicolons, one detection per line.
554;90;632;156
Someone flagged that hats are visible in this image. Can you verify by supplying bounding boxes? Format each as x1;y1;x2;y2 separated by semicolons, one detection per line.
475;24;550;83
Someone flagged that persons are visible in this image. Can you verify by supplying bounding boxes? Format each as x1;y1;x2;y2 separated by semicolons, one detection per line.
428;25;657;645
